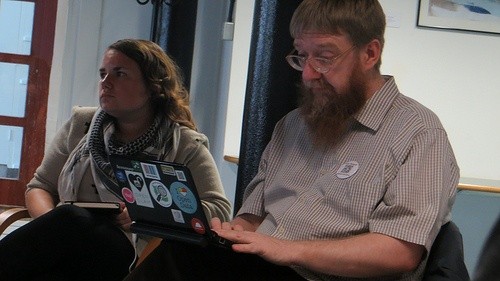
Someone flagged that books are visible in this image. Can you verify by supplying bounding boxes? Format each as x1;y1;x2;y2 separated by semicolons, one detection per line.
64;200;123;215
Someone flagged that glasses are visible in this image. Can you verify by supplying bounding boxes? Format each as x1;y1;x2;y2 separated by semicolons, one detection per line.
286;45;357;73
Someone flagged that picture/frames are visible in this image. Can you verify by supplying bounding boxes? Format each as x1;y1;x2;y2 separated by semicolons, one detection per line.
416;0;500;34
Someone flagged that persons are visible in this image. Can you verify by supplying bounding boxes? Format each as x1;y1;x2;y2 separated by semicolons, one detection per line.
0;39;232;281
121;0;460;281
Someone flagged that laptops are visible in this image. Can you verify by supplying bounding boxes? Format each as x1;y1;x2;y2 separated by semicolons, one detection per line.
109;153;240;250
